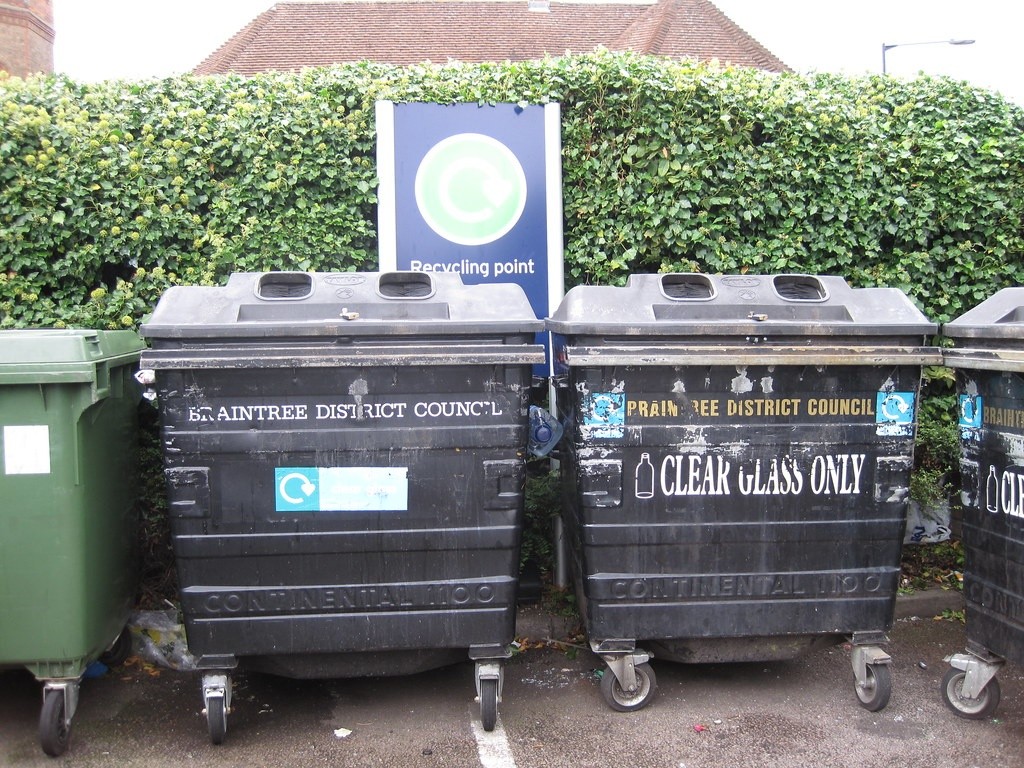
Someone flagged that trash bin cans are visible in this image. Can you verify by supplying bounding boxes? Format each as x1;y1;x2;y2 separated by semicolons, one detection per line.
135;269;545;744
0;326;146;756
545;272;938;713
940;286;1024;723
